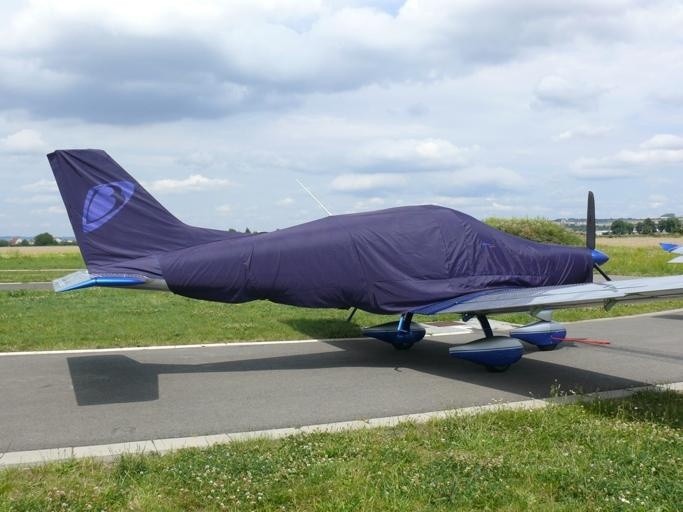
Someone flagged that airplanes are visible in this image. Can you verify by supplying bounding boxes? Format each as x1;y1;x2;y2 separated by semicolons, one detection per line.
655;237;683;267
42;147;682;371
